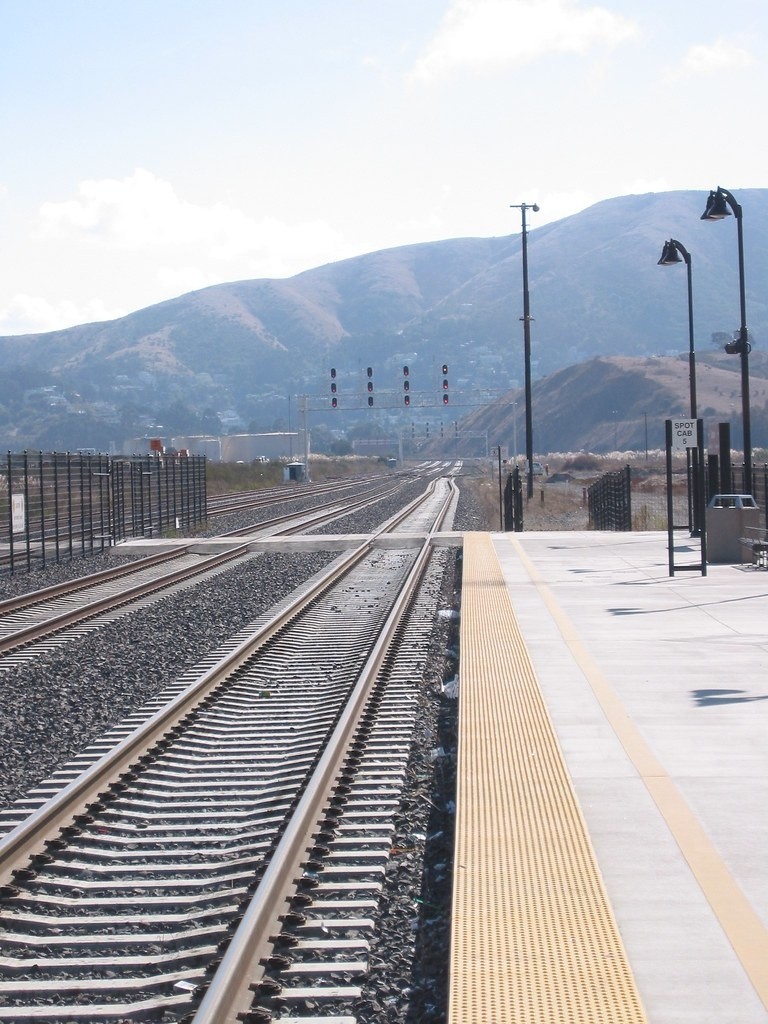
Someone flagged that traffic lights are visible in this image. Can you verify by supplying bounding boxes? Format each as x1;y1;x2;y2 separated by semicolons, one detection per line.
332;398;337;407
403;365;409;376
405;395;409;405
368;397;373;406
442;394;448;405
367;381;373;392
330;368;336;378
442;365;448;374
367;367;372;377
404;381;410;391
330;383;336;393
442;379;448;390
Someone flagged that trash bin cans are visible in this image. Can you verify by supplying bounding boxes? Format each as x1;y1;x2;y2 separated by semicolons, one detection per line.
706;494;761;564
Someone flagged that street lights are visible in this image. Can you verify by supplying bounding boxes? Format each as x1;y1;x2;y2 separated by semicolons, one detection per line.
639;411;649;462
508;203;542;498
700;186;755;508
655;237;702;536
611;410;620;452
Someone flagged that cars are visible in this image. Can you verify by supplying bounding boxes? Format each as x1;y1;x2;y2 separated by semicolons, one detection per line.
524;461;544;475
